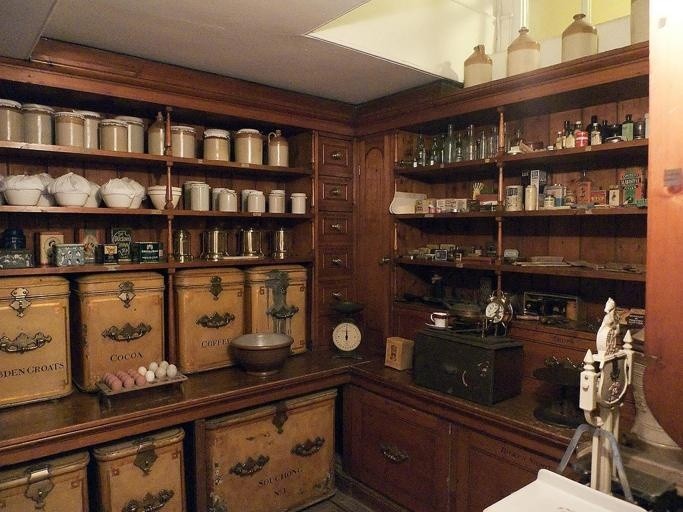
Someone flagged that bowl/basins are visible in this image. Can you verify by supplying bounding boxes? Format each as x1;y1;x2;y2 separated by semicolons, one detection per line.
229;332;294;377
3;185;181;209
448;303;481;322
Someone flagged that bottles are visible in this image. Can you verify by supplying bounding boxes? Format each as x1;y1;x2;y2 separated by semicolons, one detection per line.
543;196;554;209
525;185;536;210
412;123;526;168
560;13;598;65
463;42;493;89
266;128;289;167
505;26;541;80
555;112;651;149
146;113;166;155
630;1;648;44
576;170;592;209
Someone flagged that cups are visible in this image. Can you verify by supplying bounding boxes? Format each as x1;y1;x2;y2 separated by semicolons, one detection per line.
430;313;447;327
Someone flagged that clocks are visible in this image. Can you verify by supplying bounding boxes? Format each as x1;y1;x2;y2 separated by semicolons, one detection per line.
483;289;515;339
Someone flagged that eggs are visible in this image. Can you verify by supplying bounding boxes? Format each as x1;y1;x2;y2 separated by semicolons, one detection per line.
103;361;177;390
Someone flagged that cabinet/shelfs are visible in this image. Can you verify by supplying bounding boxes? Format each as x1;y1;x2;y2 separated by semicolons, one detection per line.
0;60;354;412
342;385;584;511
357;41;644;420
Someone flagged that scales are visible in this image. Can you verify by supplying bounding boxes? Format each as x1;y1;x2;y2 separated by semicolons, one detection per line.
331;302;364;359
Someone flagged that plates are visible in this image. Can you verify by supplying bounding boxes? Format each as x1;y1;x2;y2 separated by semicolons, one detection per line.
425;323;452;330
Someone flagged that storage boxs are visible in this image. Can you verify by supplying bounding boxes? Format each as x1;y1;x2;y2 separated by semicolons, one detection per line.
1;449;91;510
203;390;339;511
91;426;185;511
0;275;73;408
243;264;308;356
171;267;245;375
67;271;165;394
409;328;525;409
